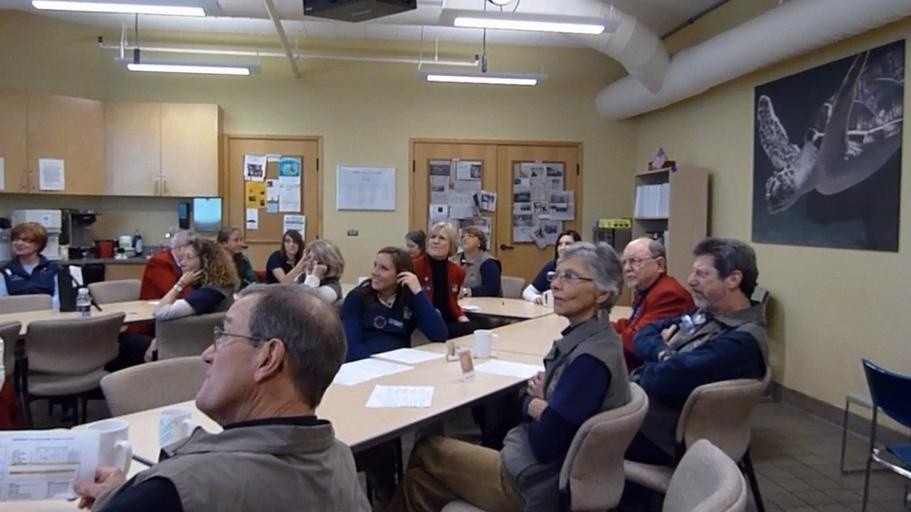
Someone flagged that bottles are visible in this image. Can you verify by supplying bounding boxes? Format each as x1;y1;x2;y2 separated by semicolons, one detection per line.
76;286;92;316
161;233;171;252
113;229;143;260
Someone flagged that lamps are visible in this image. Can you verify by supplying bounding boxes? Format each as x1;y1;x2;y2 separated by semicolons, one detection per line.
409;33;550;87
437;0;621;36
115;20;263;75
16;0;221;18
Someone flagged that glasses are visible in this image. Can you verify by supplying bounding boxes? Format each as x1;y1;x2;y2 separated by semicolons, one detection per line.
12;237;49;244
545;271;596;286
619;253;667;264
213;325;282;345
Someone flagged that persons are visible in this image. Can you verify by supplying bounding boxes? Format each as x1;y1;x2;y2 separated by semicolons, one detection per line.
391;231;770;512
1;222;58;309
338;223;504;505
104;225;344;363
73;283;373;512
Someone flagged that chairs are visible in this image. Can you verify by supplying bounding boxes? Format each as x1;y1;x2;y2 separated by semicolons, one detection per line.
860;358;911;512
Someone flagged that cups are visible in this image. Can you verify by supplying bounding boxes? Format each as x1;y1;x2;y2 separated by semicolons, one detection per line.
473;328;498;360
79;418;134;493
159;407;195;449
95;238;113;259
544;290;558;308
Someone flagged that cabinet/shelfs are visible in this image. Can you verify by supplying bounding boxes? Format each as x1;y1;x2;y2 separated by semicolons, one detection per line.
632;166;711;284
1;90;224;197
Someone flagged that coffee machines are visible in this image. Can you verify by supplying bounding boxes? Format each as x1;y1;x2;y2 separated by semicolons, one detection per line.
60;206;100;262
